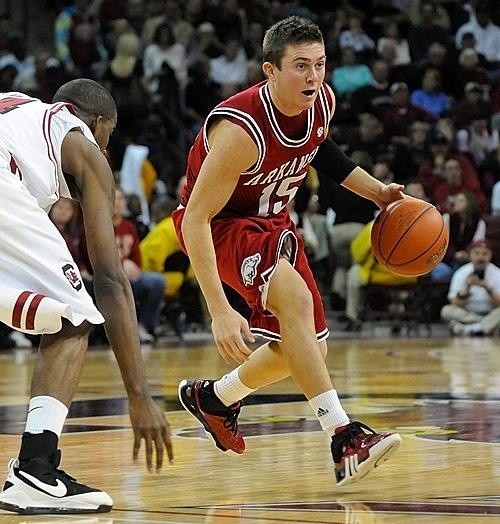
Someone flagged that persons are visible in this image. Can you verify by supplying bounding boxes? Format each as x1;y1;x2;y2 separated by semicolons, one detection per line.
0;77;177;518
0;0;499;350
171;11;441;486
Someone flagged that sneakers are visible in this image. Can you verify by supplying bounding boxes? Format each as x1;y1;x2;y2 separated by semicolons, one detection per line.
0;449;113;515
448;320;466;337
137;322;154;345
178;379;245;456
330;420;402;485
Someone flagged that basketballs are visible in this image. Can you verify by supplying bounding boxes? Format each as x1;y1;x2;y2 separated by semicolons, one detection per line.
371;198;450;279
